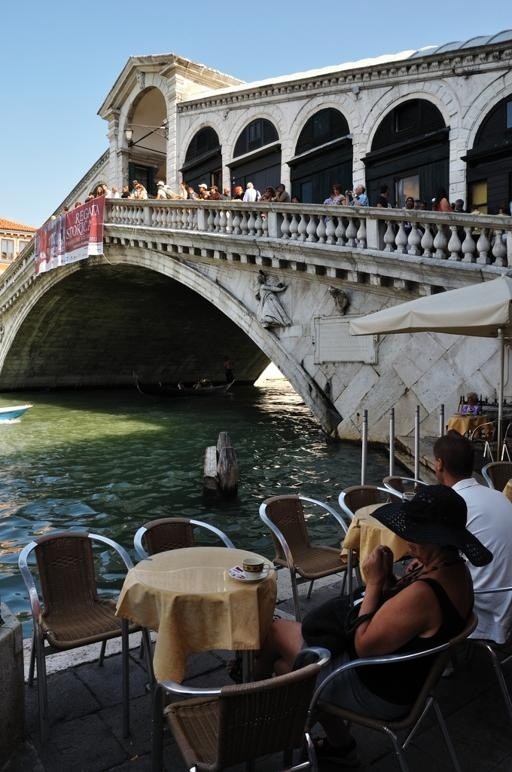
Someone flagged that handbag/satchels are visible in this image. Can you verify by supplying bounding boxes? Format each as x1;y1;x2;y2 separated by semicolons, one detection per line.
299;586;376;650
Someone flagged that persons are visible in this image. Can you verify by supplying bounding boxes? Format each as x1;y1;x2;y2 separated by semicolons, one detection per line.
221;481;495;764
460;391;484;415
404;431;512;681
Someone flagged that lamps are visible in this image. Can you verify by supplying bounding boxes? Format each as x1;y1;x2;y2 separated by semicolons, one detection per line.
121;115;170;147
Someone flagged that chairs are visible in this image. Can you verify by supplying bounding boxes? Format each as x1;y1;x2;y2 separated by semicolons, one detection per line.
383;474;429;501
310;615;483;770
146;646;330;768
404;636;512;751
469;419;512;463
337;484;405;594
134;518;233;561
259;494;357;623
18;525;138;743
482;458;512;490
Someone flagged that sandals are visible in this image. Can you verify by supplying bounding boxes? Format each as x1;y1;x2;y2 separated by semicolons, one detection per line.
303;734;361;767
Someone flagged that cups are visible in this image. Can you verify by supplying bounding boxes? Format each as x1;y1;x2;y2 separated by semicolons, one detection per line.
243;557;270;577
403;491;417;505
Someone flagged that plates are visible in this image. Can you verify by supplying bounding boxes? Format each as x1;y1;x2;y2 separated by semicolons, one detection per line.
228;566;272;583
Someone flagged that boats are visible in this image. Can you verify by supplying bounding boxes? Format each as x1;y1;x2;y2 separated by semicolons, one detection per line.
0;404;34;420
129;378;236;398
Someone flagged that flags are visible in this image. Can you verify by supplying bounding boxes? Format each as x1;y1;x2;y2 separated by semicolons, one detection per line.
33;192;109;276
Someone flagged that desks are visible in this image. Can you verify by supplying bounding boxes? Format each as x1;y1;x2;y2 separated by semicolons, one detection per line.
113;543;277;689
339;502;416;587
448;414;493;442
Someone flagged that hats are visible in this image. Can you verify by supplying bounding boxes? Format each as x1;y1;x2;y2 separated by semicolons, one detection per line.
156;180;166;187
197;183;208;190
367;482;495;570
